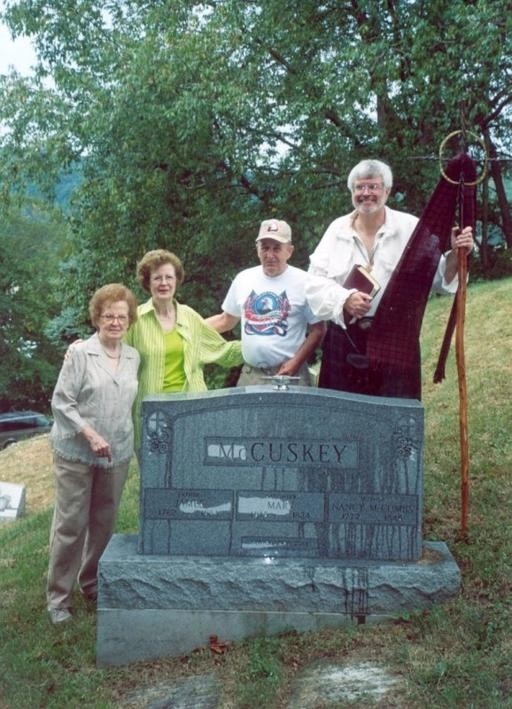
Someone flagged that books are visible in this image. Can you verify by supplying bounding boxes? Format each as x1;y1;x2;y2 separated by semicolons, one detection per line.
341;263;381;323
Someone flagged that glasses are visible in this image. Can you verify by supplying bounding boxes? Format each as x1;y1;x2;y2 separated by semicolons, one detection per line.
98;313;128;321
353;182;384;194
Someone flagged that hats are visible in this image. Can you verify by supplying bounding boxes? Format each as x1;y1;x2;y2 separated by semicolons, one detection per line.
254;218;292;244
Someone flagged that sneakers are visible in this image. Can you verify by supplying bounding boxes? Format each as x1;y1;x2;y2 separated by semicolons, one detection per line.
48;608;73;626
83;594;97;611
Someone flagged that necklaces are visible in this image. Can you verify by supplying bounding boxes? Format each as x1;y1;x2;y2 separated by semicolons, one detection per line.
102;343;121;359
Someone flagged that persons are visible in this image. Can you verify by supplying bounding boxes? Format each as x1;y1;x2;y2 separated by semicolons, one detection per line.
303;158;473;398
45;283;141;630
202;219;327;388
62;249;244;470
